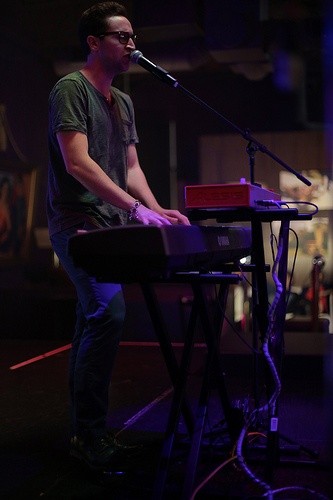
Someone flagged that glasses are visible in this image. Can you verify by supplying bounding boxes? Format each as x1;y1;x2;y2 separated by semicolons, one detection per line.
88;31;137;44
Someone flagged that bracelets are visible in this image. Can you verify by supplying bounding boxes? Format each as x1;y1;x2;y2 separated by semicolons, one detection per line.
128;199;141;222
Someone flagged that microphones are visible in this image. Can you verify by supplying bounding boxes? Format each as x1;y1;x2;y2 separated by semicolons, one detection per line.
131;51;179;88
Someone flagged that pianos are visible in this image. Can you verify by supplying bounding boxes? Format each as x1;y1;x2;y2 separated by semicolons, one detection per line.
70;223;251;281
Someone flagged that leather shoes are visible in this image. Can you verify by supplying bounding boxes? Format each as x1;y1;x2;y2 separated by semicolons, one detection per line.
70;431;135;486
69;431;146;459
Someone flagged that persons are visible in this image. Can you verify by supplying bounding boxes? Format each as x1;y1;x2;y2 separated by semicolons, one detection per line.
46;3;193;489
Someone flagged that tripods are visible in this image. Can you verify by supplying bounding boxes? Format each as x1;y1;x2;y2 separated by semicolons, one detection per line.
201;141;323;479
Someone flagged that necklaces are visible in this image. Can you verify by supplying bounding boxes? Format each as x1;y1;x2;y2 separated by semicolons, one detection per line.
80;70;111;101
